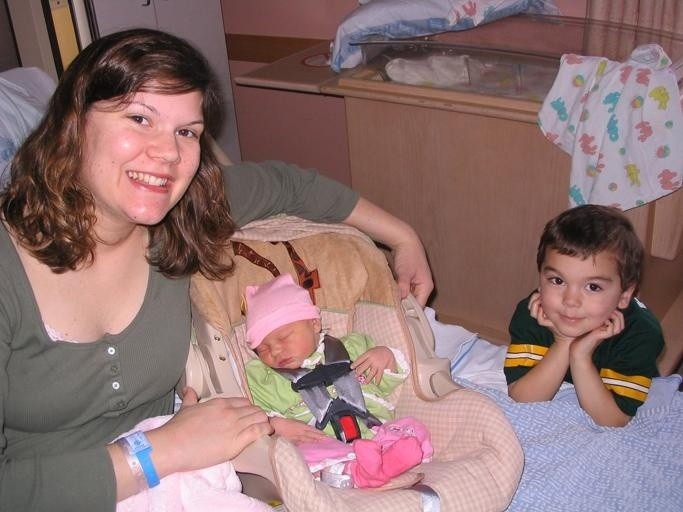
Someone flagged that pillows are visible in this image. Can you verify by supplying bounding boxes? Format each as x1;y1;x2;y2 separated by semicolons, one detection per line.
329;0;561;72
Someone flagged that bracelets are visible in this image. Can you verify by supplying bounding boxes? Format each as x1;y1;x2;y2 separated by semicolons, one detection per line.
116;430;161;495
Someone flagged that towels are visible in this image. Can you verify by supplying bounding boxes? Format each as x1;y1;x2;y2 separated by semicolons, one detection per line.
537;42;682;215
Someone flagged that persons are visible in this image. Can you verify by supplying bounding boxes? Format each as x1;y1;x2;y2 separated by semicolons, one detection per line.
0;29;435;511
243;274;434;488
503;202;663;428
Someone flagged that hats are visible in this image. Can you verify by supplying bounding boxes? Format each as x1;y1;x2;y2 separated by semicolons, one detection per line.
243;272;322;351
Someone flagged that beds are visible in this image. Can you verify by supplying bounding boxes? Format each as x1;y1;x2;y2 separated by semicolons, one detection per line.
0;63;681;512
